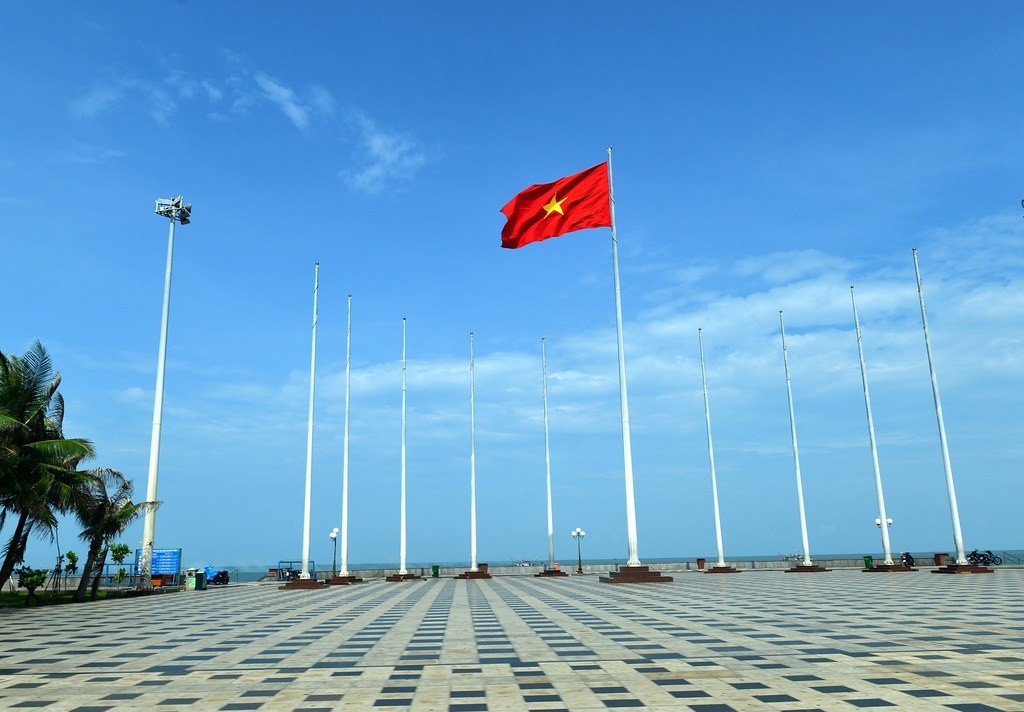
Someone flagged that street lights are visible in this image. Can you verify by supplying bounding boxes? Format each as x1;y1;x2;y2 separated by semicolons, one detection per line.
328;527;343;579
571;528;588;575
136;195;194;588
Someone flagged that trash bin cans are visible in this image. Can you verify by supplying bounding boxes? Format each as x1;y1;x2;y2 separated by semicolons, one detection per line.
432;565;439;577
935;554;949;566
186;571;196;591
863;555;874;569
697;559;705;569
478;563;487;573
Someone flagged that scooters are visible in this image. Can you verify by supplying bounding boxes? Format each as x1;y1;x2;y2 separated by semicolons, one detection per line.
967;548;1003;566
899;551;917;568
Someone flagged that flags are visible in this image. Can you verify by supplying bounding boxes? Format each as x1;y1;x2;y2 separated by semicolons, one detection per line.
501;160;612;249
